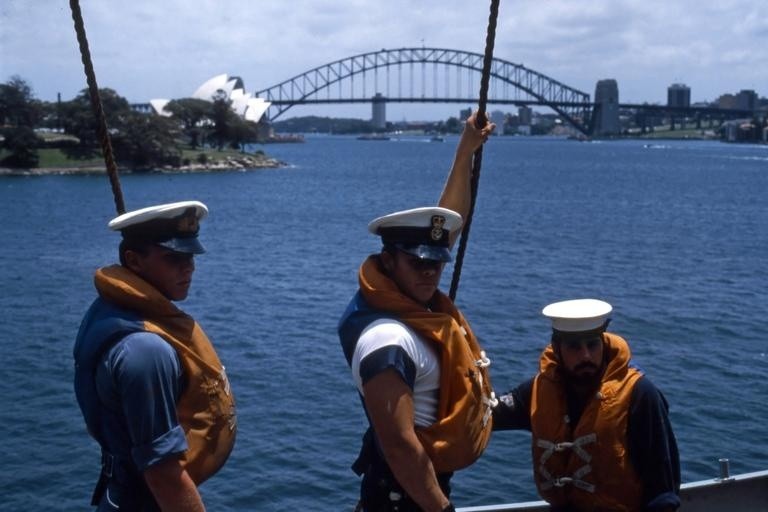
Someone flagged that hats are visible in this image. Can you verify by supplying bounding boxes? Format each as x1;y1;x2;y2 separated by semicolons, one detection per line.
109;200;209;254
368;206;463;262
542;298;613;338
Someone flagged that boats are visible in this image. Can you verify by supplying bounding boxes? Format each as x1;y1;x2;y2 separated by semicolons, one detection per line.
252;132;308;145
431;136;446;143
357;134;393;141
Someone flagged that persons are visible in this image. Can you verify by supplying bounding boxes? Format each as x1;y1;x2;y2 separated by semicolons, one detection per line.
338;109;495;511
494;299;681;512
74;201;235;512
